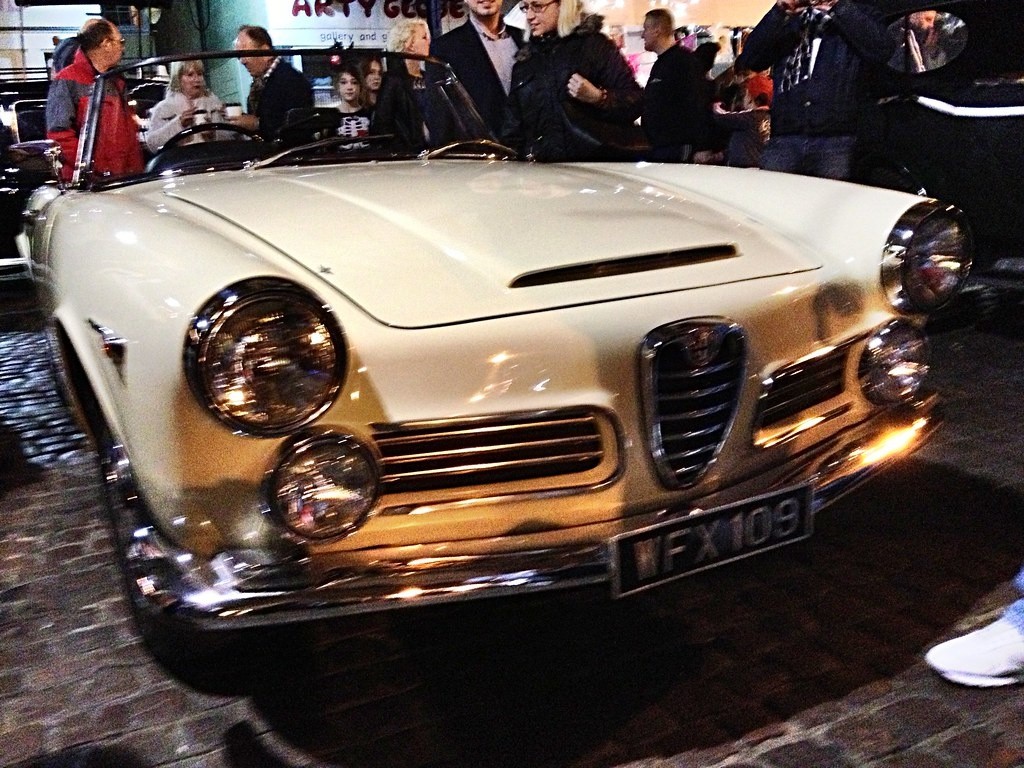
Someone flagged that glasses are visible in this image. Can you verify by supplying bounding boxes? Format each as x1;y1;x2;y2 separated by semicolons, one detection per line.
108;38;126;46
520;0;556;13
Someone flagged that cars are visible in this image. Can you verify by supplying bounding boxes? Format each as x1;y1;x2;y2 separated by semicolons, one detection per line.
14;47;974;662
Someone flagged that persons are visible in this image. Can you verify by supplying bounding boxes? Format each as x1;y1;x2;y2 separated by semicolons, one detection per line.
924;566;1024;689
220;25;316;141
331;1;896;194
46;19;144;184
146;60;243;155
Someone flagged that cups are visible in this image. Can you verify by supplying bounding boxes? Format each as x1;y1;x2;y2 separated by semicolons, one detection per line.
191;110;208;134
224;103;243;120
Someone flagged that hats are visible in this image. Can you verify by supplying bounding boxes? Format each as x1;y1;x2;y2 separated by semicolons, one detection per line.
745;75;774;103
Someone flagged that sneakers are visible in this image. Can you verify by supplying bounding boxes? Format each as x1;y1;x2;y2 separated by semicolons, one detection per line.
924;616;1024;687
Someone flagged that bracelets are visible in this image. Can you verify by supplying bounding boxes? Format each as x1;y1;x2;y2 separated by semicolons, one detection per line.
595;88;608;106
254;118;260;130
